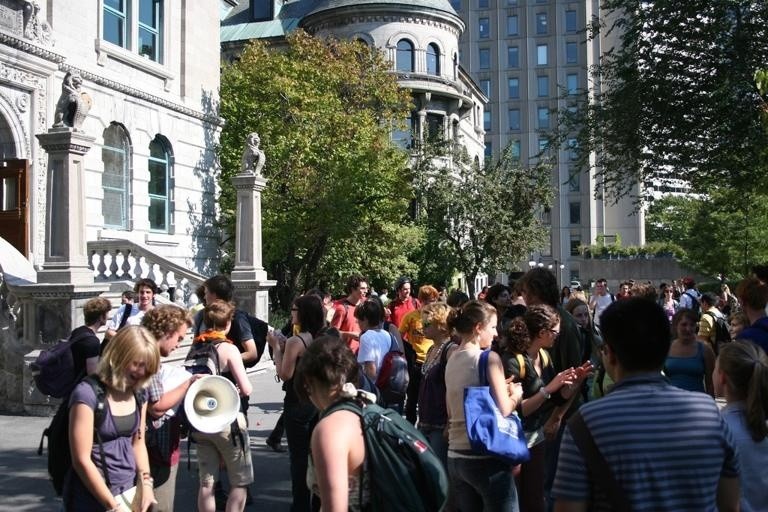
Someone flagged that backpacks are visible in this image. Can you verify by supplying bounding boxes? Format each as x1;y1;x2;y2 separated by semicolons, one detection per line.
684;290;703;314
38;373;142;498
176;339;232;434
232;309;268;368
318;397;450;512
30;332;99;398
704;311;732;356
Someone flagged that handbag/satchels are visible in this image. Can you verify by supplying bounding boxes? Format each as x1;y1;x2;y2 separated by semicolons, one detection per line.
375;330;410;405
462;351;532;464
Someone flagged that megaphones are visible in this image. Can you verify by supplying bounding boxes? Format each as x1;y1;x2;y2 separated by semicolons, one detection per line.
183;374;241;434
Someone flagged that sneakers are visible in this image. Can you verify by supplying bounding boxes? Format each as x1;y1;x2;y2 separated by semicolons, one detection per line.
266;439;287;453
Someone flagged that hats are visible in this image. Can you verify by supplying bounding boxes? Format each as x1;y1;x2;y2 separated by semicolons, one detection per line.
395;277;411;293
485;284;511;302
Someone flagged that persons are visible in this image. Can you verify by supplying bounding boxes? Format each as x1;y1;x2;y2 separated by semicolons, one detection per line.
49;262;768;512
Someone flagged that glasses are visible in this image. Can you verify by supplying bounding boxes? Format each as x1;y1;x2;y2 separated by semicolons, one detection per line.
542;327;560;341
422;320;434;328
